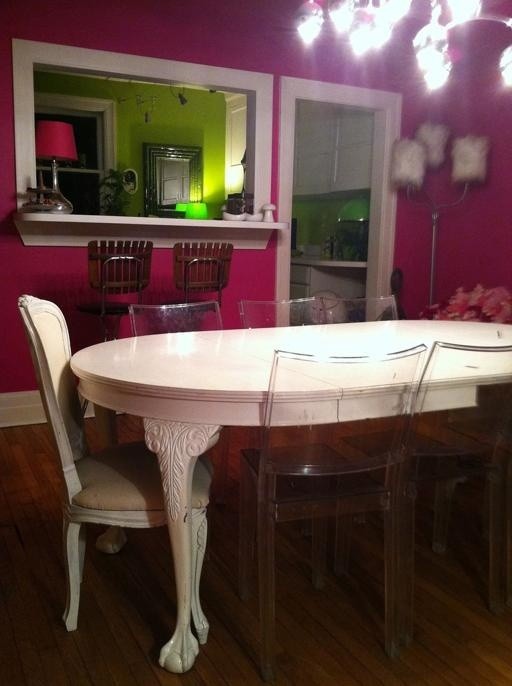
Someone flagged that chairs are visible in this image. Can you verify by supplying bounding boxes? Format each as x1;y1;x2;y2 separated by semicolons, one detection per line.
18;241;511;684
16;240;511;686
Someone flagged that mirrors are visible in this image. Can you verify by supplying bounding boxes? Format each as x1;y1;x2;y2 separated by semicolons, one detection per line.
145;143;204;217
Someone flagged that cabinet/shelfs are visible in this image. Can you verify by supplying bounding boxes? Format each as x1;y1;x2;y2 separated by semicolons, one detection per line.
222;90;373;200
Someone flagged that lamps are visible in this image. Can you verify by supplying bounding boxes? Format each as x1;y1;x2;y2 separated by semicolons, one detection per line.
35;120;80;215
383;120;489;307
